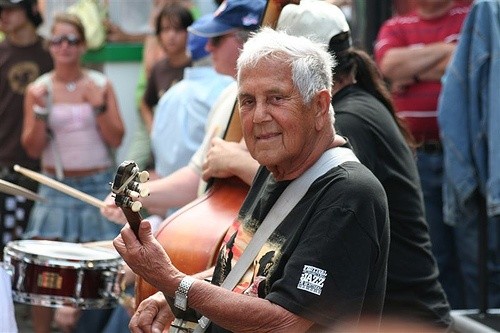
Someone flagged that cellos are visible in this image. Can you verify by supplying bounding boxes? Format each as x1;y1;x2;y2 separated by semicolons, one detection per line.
132;0;304;311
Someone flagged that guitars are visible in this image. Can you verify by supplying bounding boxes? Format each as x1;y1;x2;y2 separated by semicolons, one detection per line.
112;162;216;333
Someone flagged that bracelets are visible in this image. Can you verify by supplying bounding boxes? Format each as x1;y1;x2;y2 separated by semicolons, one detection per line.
412;75;420;83
92;104;107;114
32;104;49;119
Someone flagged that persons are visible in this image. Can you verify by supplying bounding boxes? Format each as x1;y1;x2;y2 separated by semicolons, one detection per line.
113;26;390;333
0;0;127;333
373;0;500;333
102;1;452;333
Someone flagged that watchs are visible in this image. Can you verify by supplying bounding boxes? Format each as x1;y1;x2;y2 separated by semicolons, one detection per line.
173;276;196;312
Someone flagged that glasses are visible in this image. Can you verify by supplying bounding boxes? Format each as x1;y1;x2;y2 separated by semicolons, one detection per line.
47;34;83;45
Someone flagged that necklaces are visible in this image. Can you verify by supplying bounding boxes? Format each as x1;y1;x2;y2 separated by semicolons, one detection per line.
66;82;78;92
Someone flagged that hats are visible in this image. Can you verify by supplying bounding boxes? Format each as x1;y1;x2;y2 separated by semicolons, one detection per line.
0;0;43;27
276;1;352;52
186;0;265;38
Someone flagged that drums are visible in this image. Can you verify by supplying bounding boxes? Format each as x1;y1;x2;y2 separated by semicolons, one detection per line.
2;240;122;310
